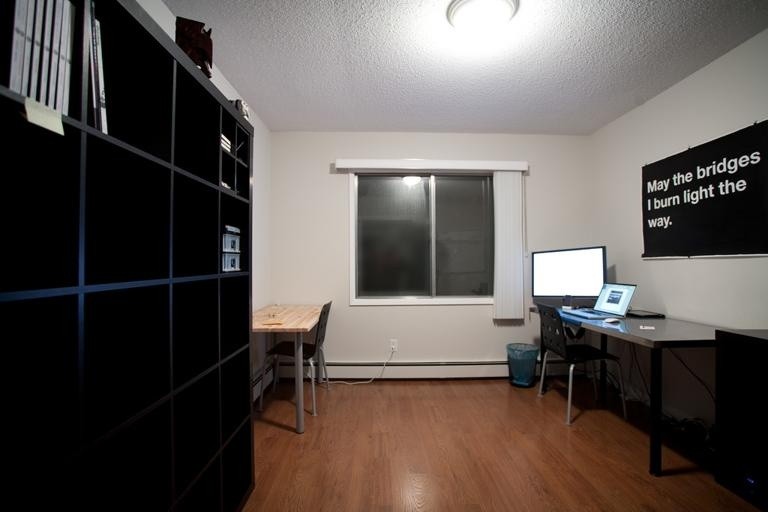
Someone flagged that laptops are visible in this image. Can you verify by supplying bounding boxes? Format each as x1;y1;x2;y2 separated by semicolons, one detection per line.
562;282;637;320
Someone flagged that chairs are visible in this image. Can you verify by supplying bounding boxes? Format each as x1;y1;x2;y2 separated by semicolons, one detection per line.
536;302;628;428
258;300;332;416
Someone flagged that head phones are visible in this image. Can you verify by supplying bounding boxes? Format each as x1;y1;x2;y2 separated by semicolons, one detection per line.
564;320;585;339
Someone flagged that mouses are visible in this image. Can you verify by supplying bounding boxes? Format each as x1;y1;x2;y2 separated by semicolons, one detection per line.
605;318;620;323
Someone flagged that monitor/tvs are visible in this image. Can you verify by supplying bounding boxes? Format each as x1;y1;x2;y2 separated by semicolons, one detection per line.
532;246;606;310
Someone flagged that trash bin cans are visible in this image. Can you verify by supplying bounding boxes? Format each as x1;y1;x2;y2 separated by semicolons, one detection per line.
506;343;538;386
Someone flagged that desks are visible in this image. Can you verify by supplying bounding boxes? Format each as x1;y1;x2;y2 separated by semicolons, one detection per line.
250;303;325;436
530;303;728;477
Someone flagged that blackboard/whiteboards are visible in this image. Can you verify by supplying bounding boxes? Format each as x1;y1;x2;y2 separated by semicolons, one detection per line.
641;120;767;261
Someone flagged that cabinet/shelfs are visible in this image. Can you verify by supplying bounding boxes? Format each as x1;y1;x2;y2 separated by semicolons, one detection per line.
0;0;256;512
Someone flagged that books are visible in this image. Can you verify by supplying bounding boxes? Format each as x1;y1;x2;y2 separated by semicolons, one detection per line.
9;0;116;136
221;133;231;152
